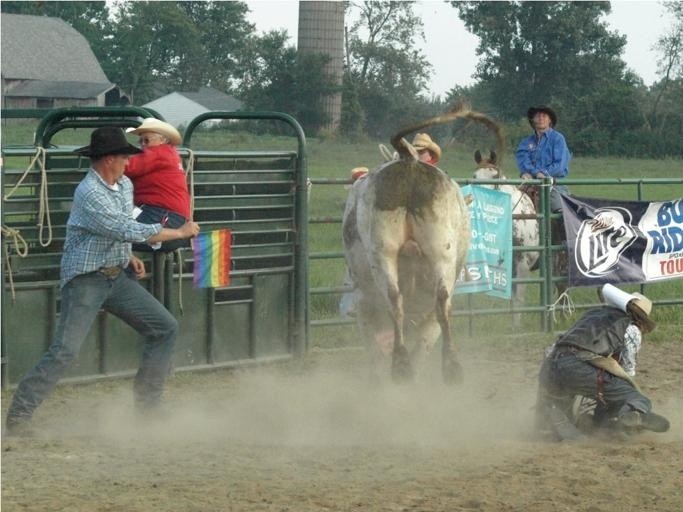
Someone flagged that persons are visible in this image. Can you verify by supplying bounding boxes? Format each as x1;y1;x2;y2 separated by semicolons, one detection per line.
122;117;190;252
412;133;441;167
0;128;201;439
531;282;668;442
514;105;572;259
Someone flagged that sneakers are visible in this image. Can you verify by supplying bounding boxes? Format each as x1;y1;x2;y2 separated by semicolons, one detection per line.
618;410;670;433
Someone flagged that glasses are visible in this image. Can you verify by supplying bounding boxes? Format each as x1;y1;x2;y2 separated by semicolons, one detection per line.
137;137;156;143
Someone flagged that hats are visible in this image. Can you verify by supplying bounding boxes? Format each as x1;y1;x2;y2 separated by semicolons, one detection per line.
125;117;182;147
411;133;441;160
72;127;145;157
527;105;557;129
625;292;657;334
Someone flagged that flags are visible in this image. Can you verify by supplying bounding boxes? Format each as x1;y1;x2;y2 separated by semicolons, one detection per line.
192;229;229;288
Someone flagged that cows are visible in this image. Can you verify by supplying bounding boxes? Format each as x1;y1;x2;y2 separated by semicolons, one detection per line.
339;105;503;398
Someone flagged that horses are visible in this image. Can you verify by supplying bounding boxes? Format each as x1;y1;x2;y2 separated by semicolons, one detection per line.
469;149;543;334
